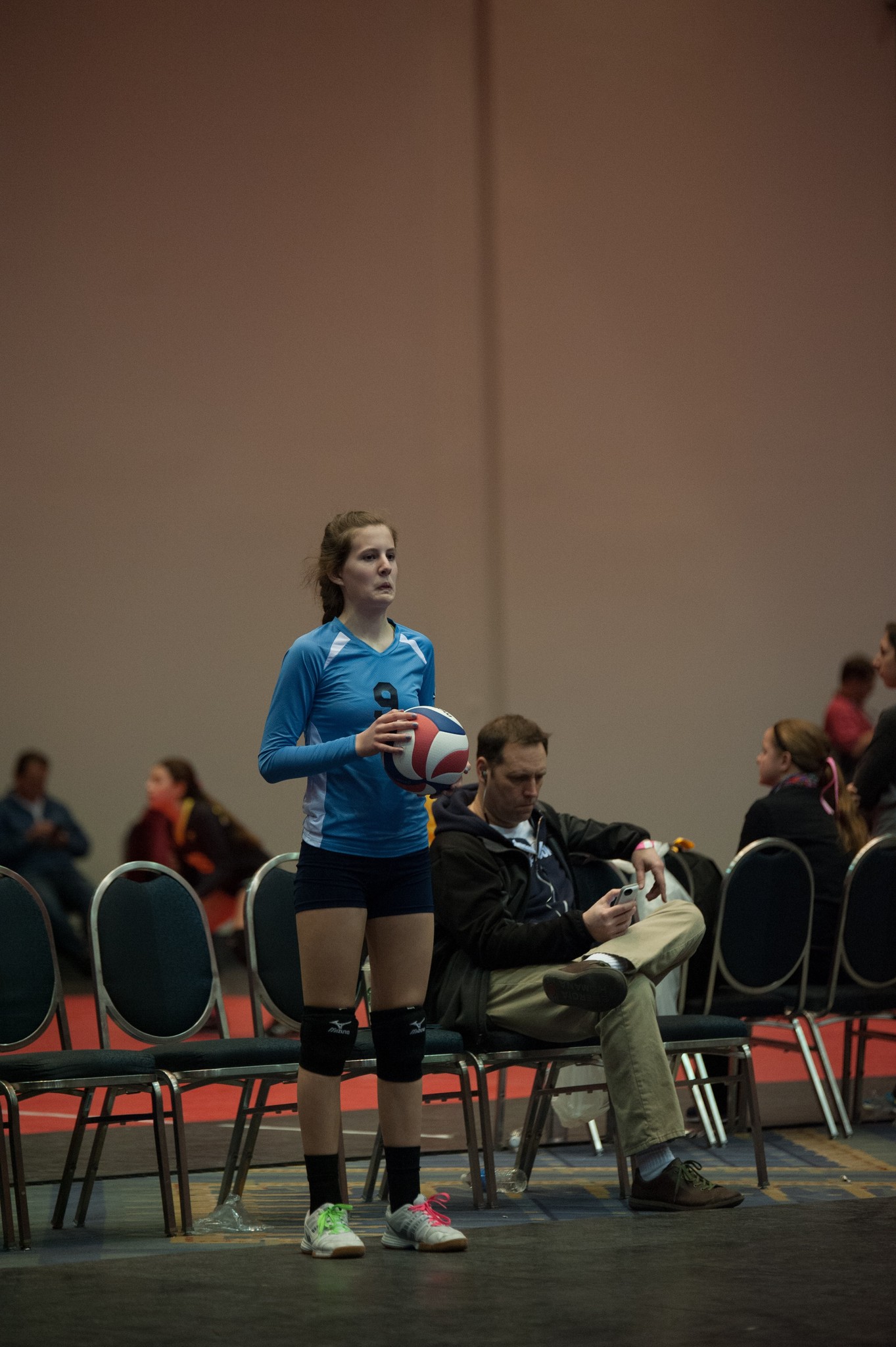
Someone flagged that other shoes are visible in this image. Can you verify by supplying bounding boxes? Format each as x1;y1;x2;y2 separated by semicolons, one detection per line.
686;1098;730;1122
339;919;543;1013
629;1157;747;1210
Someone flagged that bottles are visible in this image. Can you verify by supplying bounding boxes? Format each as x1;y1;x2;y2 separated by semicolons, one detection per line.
460;1167;527;1193
508;1128;525;1153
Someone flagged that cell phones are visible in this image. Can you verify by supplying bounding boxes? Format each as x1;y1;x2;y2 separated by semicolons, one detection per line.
614;882;639;907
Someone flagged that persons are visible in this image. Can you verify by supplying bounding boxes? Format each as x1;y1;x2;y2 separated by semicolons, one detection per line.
1;626;896;1214
257;510;469;1258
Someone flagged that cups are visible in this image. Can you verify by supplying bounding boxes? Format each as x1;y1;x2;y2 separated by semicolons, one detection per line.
362;955;373;1026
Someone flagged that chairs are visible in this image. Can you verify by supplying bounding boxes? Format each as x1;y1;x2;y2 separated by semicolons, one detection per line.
0;835;896;1259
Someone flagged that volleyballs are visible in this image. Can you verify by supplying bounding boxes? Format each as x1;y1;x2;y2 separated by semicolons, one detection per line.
381;705;471;795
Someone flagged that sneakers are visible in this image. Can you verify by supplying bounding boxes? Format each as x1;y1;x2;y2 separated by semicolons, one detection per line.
301;1202;366;1259
381;1194;468;1251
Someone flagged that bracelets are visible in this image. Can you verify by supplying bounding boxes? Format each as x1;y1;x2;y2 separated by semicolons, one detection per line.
635;840;655;850
